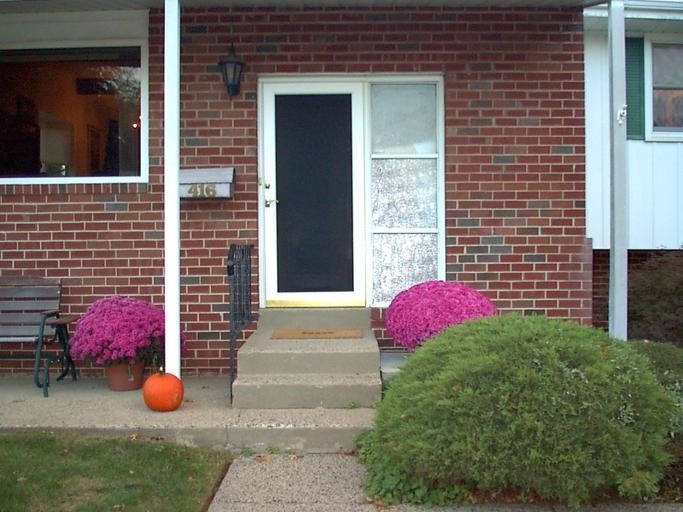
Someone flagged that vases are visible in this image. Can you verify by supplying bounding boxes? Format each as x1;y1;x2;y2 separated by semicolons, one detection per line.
106;358;146;391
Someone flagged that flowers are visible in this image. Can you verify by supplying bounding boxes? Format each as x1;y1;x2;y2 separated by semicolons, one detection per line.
66;296;187;364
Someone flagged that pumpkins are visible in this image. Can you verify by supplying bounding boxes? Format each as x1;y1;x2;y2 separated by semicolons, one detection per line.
143;366;184;411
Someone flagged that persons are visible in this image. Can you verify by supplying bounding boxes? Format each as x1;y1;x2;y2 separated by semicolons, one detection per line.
106;119;135;176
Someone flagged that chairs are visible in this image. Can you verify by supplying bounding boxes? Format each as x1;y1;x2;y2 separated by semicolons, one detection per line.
0;275;79;388
